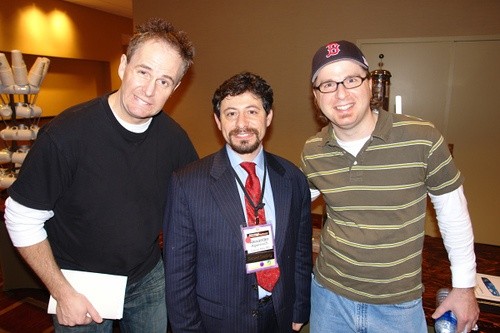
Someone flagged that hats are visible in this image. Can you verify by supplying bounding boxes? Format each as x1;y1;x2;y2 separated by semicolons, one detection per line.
312;40;369;83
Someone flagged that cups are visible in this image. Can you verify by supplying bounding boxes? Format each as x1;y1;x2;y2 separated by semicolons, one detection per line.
0;50;50;187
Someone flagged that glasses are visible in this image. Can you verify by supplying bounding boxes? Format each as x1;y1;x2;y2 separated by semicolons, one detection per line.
313;76;368;93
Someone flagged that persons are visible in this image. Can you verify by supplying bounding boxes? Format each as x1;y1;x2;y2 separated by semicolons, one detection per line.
3;17;200;333
163;71;313;333
299;40;480;333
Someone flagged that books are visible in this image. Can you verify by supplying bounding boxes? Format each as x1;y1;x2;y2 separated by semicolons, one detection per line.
474;272;500;306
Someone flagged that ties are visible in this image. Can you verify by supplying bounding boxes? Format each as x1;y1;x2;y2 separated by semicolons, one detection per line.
239;162;280;292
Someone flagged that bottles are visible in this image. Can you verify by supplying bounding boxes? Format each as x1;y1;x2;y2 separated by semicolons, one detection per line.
434;288;457;333
